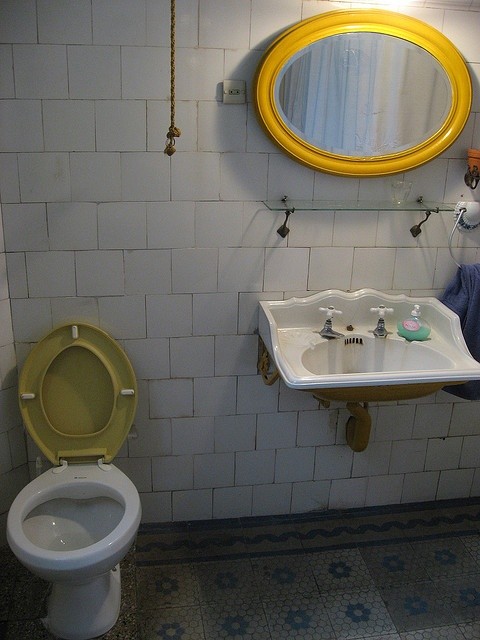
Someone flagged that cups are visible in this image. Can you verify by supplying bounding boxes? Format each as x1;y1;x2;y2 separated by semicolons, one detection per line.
391;179;414;208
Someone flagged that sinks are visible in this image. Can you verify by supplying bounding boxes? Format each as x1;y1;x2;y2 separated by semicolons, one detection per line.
293;331;457;381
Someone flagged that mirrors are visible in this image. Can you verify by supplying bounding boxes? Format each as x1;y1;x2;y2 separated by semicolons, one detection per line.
251;4;475;179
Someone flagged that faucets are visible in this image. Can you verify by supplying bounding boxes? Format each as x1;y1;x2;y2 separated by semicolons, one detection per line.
315;305;344;342
369;305;394;341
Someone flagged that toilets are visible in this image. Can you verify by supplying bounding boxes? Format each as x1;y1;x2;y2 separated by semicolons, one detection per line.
6;317;143;639
